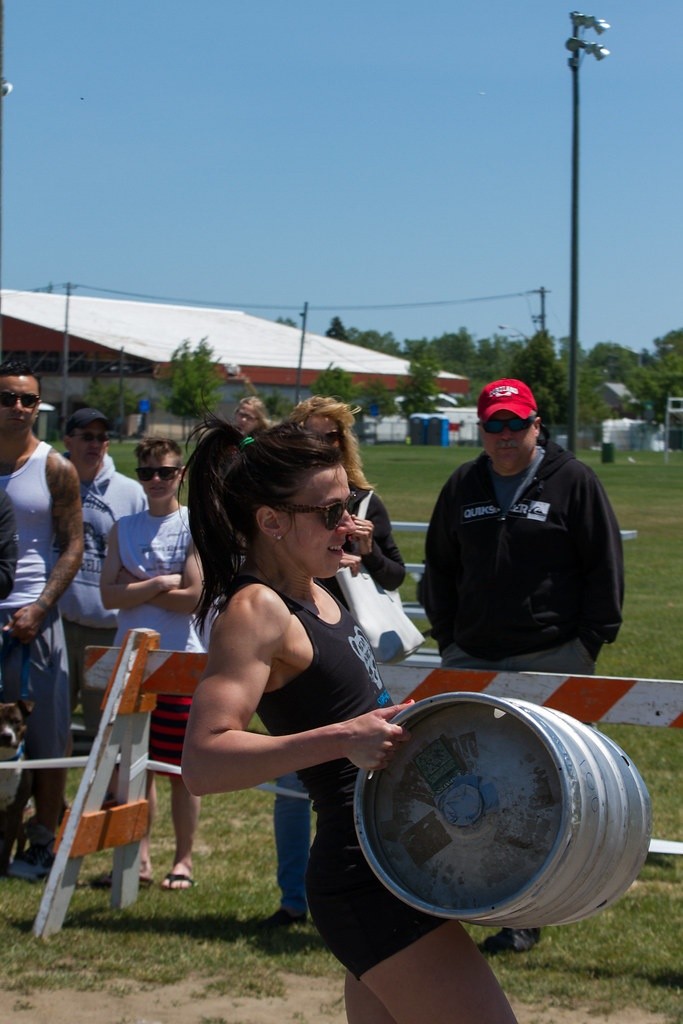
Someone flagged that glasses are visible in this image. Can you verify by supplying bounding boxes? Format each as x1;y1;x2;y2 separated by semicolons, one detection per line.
71;432;110;443
0;391;41;407
259;488;357;531
237;411;257;423
476;416;537;435
322;428;345;444
133;465;182;481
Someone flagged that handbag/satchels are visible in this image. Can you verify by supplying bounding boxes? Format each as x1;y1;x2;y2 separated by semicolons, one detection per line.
336;485;425;666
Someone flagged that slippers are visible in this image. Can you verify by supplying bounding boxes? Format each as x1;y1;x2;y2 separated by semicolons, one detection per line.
107;867;153;888
158;873;195;892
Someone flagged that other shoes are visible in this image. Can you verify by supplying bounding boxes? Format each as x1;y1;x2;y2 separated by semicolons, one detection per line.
481;928;538;954
259;908;307;929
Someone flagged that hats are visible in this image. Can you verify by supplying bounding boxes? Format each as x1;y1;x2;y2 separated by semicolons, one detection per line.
476;378;538;420
63;408;111;433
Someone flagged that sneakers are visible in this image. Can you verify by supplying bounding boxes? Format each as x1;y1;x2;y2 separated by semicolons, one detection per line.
8;844;57;883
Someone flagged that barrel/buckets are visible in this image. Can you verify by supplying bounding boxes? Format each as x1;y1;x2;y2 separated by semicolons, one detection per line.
603;442;614;463
354;691;651;930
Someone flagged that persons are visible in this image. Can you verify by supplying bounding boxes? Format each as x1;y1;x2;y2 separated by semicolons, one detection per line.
100;437;220;891
234;395;268;438
0;359;85;881
59;408;150;756
416;379;627;675
261;395;407;931
179;418;519;1024
